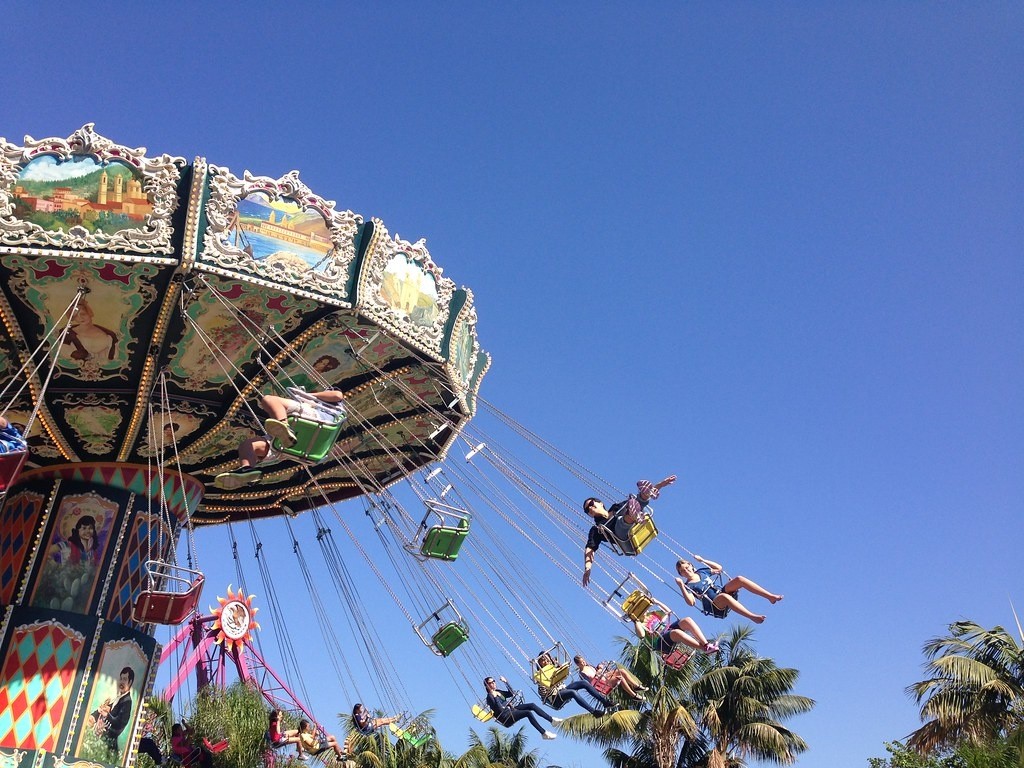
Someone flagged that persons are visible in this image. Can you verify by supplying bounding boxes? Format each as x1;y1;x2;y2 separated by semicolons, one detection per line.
297;719;347;762
583;474;677;587
138;737;170;767
171;718;207;768
268;709;309;761
536;651;620;719
215;387;343;490
630;597;719;656
0;414;28;454
484;675;563;739
675;554;784;624
574;654;649;702
353;703;402;734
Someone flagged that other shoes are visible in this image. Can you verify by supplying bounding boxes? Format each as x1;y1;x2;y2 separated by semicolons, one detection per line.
296;747;307;752
593;710;605;719
603;699;619;708
335;752;346;762
394;712;402;723
542;730;557;739
637;480;660;500
551;717;564;728
297;754;309;761
628;493;645;523
699;643;719;654
709;641;718;646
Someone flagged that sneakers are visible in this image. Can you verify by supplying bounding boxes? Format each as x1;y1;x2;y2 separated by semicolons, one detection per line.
215;466;261;490
265;419;298;450
634;684;649;692
632;693;646;702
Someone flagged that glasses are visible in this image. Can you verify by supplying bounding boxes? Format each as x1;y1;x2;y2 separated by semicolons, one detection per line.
489;680;495;684
584;499;594;513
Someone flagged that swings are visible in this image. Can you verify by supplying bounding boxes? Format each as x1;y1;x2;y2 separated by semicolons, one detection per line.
0;274;729;768
